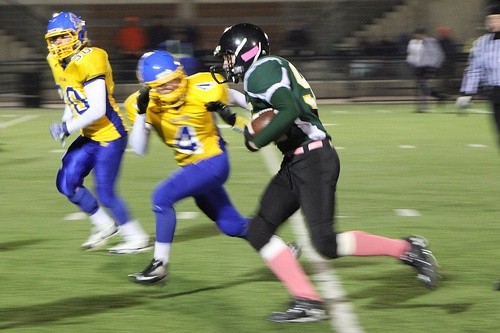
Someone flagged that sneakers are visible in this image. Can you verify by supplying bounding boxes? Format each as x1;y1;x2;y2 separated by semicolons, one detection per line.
265;296;329;323
400;235;441;288
287;241;303;259
127;258;168;284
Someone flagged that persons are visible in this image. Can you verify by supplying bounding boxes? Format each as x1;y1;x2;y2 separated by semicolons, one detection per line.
455;5;500;134
115;17;144;54
204;23;440;323
124;50;302;285
45;11;154;253
407;26;456;112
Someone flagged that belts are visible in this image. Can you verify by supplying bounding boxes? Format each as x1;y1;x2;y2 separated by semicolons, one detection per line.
293;139;332;155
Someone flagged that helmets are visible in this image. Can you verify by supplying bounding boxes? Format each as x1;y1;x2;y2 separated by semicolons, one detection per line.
46;11;88;61
213;23;270;84
136;48;188;110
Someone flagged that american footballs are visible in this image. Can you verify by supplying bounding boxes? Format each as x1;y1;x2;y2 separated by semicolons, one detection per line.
244;107;280;140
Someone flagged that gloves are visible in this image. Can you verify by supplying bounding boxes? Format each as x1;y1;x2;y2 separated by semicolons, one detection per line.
456;94;472;107
204;101;236;126
136;85;151;115
243;125;258;152
49;122;70;148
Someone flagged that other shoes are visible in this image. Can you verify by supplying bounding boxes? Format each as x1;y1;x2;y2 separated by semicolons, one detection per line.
107;235;153;254
81;220;119;251
